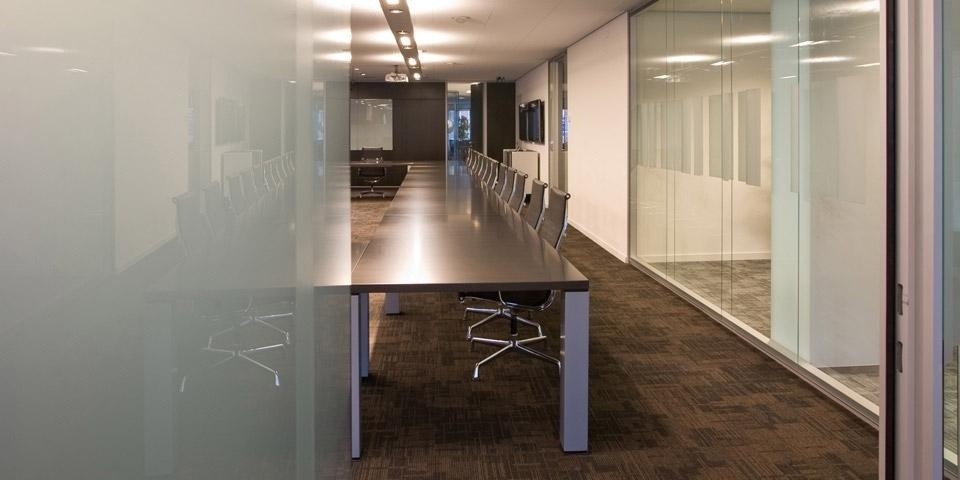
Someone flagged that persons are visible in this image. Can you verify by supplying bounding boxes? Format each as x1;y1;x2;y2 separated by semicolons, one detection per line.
447;119;454;161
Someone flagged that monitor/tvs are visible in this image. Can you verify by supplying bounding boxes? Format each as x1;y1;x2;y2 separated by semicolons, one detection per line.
519;104;528;140
528;99;542;143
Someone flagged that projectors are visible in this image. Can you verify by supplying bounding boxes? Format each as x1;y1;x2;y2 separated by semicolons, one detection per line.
385;74;408;83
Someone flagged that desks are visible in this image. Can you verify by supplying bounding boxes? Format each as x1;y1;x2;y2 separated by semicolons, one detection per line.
115;175;295;477
350;160;590;459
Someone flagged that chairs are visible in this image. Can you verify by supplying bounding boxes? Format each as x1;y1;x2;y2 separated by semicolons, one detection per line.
357;147;387;199
466;147;493;184
499;167;518;203
494;163;508;194
463;178;549;341
240;150;297;210
508;170;529;213
457;186;571;381
